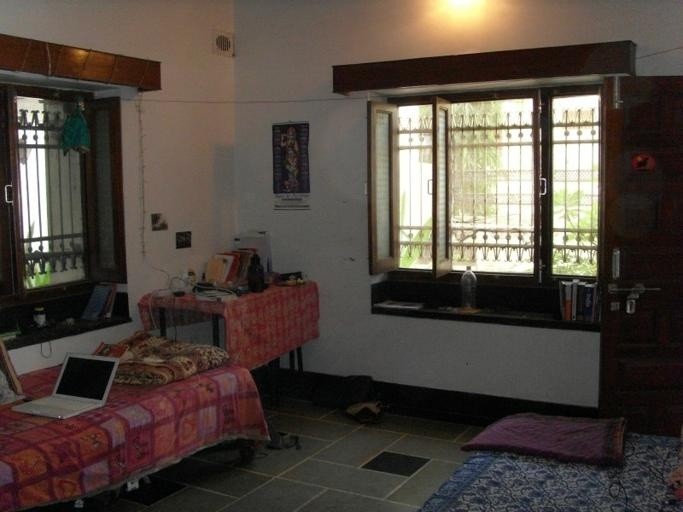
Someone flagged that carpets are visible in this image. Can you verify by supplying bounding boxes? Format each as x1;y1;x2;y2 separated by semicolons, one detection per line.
413;416;682;510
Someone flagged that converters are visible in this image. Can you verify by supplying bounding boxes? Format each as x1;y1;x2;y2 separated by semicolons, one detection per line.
10;352;121;420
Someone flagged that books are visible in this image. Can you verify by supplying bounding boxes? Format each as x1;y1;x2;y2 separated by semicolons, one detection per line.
202;230;273;292
0;334;16;340
556;278;599;324
0;318;21;337
449;306;482;314
96;281;118;318
79;283;116;322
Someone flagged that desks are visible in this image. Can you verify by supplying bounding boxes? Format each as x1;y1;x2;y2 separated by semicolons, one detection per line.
145;282;317;377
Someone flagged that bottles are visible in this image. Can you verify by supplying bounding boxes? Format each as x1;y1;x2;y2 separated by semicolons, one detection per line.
247;254;265;293
33;307;47;337
461;265;478;309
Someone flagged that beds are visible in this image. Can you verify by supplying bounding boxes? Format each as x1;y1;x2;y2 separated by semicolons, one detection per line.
0;360;253;511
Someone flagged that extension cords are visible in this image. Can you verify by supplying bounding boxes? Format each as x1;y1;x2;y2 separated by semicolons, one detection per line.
153;288;189;298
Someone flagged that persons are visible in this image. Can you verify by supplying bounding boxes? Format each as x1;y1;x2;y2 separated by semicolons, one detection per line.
373;299;427;311
278;127;300;192
